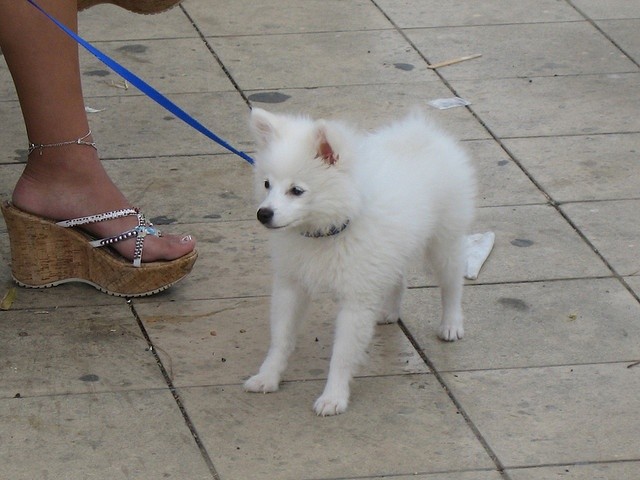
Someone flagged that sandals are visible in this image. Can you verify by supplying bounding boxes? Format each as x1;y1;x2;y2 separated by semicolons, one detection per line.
1;199;198;297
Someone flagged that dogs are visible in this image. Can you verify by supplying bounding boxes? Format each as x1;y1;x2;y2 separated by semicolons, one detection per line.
242;109;475;416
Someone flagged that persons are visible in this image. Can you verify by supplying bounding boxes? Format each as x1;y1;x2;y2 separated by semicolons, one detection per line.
0;0;198;300
